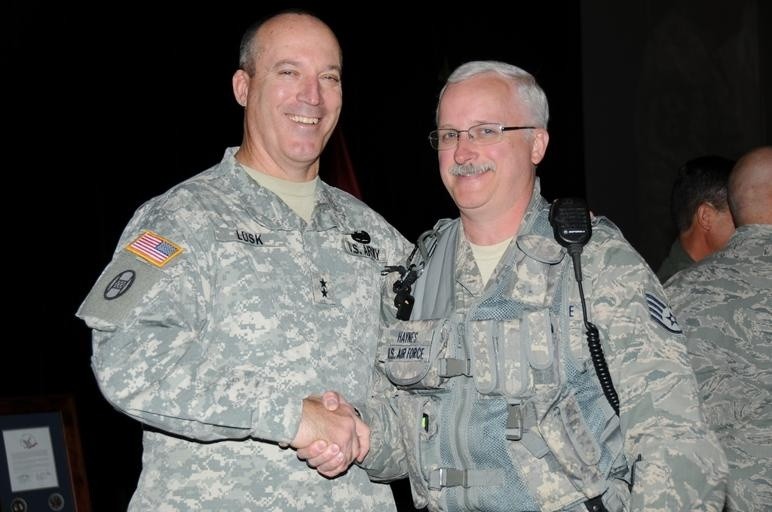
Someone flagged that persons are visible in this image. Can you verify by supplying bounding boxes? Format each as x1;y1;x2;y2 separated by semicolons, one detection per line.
275;57;733;512
72;6;423;510
661;141;772;512
651;151;740;288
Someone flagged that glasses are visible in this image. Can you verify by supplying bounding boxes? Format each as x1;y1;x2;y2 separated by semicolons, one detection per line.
427;123;541;151
